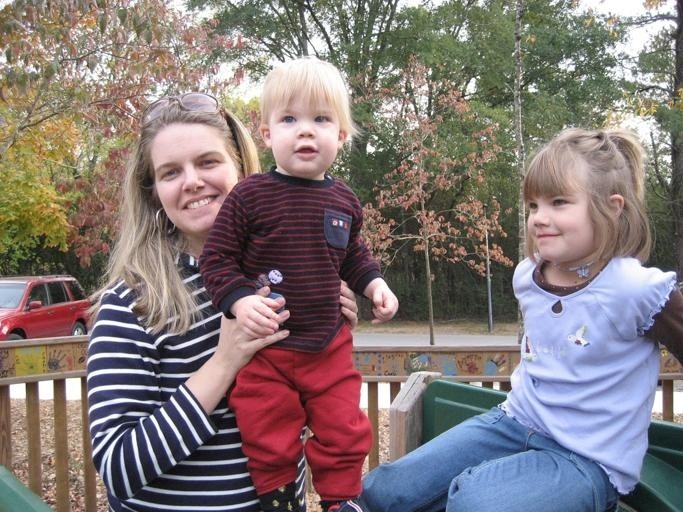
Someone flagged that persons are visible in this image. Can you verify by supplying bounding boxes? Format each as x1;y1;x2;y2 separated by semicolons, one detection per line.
84;90;360;511
359;126;683;512
197;57;400;512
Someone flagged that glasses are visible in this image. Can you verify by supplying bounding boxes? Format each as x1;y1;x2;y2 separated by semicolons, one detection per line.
137;92;229;131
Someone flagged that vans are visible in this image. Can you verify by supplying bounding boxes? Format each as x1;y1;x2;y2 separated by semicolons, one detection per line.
0;275;90;340
